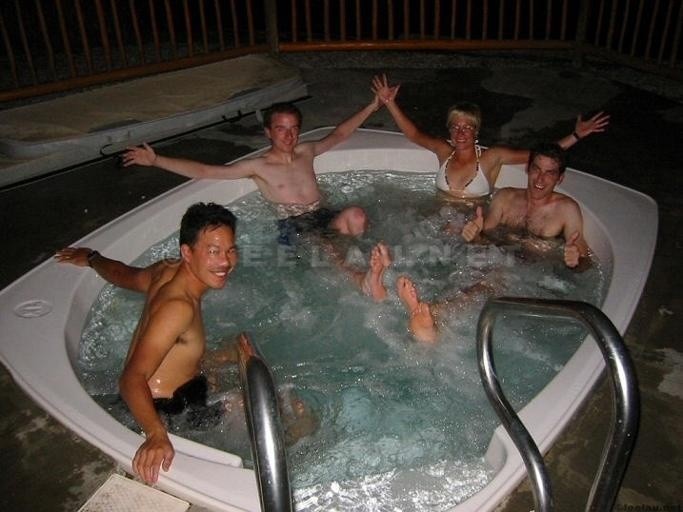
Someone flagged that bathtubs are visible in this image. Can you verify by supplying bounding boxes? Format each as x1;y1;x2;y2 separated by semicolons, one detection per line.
0;125;659;511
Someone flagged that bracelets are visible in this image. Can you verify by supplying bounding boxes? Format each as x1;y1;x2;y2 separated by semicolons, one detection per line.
570;130;581;143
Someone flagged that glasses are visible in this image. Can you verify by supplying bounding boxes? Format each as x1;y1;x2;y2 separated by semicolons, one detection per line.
449;125;476;132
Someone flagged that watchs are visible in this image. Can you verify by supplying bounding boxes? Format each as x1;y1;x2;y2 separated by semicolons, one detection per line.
89;250;103;268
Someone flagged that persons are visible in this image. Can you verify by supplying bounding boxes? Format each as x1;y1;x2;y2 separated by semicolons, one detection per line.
397;141;595;347
119;80;397;309
372;72;611;239
53;196;317;485
